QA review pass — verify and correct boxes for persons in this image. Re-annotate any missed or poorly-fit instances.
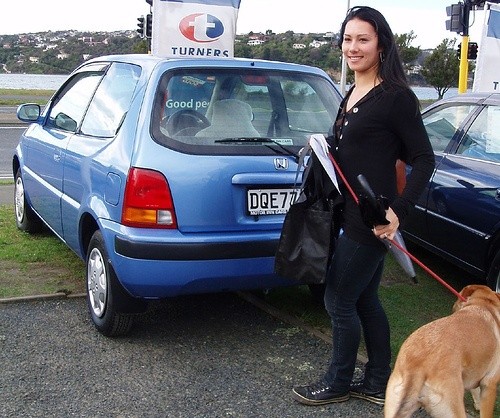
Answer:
[290,4,436,407]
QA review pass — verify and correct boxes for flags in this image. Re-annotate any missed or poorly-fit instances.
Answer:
[468,2,500,112]
[150,0,242,121]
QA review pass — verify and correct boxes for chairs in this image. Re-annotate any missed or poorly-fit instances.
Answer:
[194,98,260,140]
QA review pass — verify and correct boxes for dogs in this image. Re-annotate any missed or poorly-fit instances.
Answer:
[385,285,500,418]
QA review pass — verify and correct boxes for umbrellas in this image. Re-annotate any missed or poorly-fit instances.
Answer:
[356,174,422,285]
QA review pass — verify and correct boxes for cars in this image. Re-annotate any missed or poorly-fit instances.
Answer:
[388,94,500,297]
[11,53,345,335]
